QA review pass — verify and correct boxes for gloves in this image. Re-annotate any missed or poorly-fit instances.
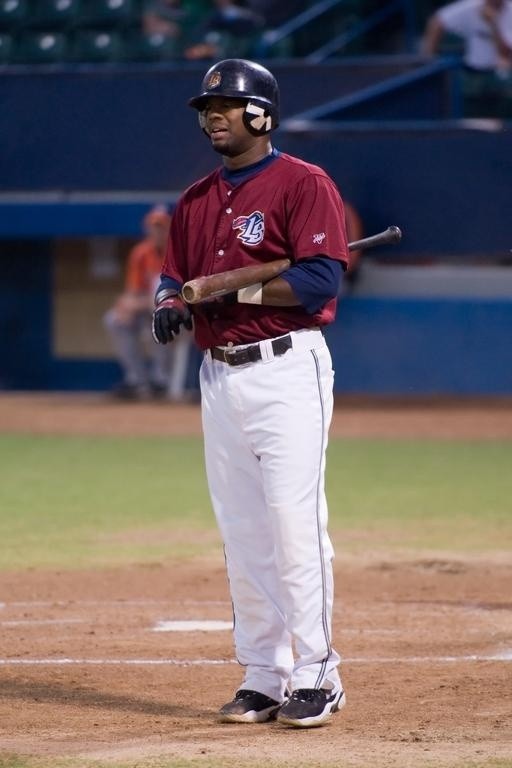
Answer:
[150,296,194,344]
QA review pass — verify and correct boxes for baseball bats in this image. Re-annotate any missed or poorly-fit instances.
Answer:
[181,225,401,304]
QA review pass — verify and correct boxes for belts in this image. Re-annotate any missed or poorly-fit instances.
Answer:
[209,334,292,365]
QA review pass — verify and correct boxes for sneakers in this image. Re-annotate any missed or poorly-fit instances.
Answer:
[277,685,346,727]
[219,686,290,727]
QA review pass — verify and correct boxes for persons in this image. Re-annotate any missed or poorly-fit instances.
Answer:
[98,200,200,409]
[131,1,238,60]
[148,55,350,730]
[415,0,512,81]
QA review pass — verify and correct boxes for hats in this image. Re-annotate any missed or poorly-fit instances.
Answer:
[145,209,172,226]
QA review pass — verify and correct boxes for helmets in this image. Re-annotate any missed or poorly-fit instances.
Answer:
[186,58,281,137]
